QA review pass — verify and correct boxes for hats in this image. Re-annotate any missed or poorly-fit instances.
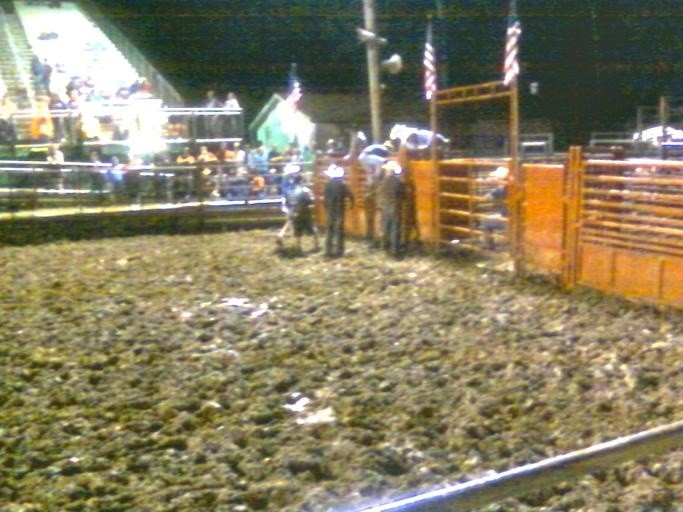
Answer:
[382,161,402,175]
[323,164,344,178]
[490,167,508,178]
[282,164,300,176]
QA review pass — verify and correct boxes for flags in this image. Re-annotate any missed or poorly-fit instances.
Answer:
[421,14,437,101]
[502,1,522,85]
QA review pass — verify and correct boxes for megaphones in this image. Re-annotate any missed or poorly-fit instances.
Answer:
[381,53,402,74]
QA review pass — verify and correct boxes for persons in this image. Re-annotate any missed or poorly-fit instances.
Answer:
[281,174,317,255]
[479,167,511,251]
[321,164,354,259]
[359,140,405,187]
[370,159,409,259]
[274,165,304,246]
[1,57,353,205]
